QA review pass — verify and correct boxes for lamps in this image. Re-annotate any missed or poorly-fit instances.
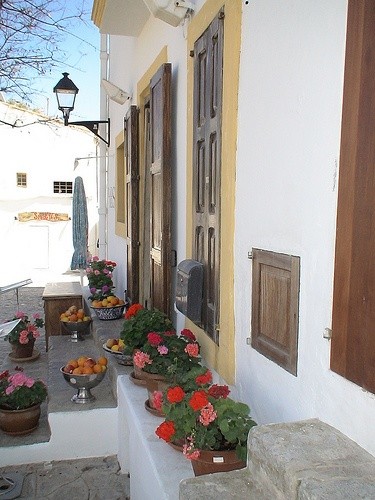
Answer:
[144,0,194,27]
[102,79,132,105]
[53,72,111,147]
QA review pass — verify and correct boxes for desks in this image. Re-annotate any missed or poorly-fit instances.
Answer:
[42,281,83,351]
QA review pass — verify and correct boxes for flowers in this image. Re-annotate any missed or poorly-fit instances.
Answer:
[120,304,257,461]
[0,366,50,409]
[3,310,45,345]
[86,256,116,299]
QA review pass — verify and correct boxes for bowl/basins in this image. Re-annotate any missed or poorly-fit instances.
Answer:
[102,342,134,366]
[89,301,128,321]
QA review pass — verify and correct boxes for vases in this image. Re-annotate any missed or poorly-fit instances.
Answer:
[169,438,184,452]
[0,404,41,435]
[129,360,162,386]
[145,377,172,417]
[191,449,245,477]
[10,338,36,358]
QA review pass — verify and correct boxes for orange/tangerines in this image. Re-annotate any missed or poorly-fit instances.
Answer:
[63,356,107,374]
[92,295,124,307]
[60,306,90,322]
[105,338,126,351]
